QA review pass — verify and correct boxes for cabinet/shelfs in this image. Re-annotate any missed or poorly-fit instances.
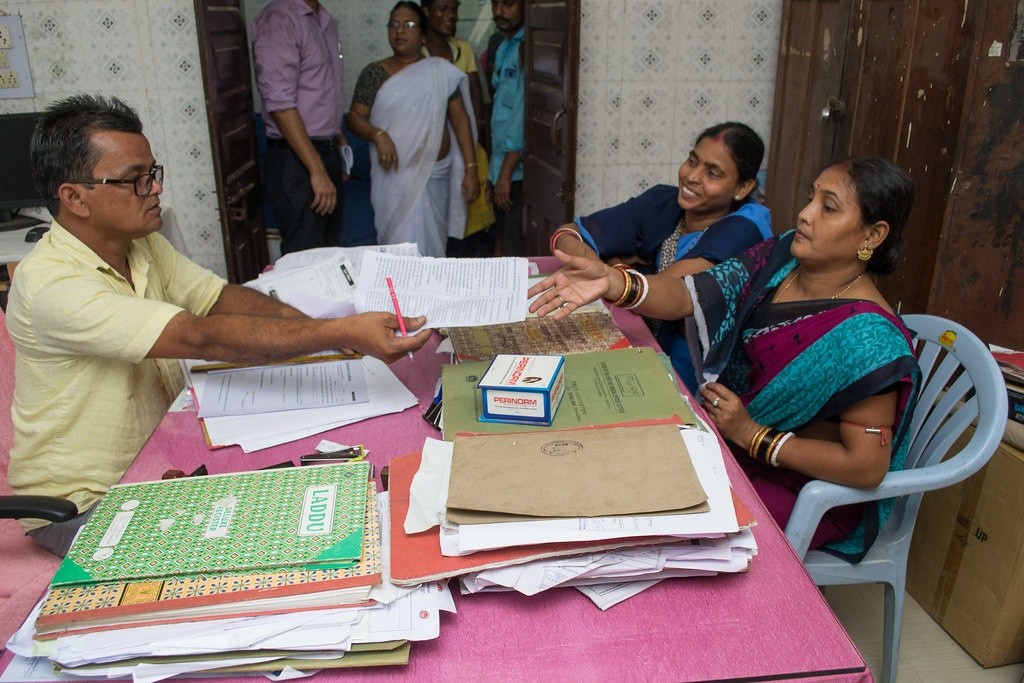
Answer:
[763,0,1024,381]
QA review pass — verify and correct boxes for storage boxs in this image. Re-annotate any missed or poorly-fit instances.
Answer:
[905,414,1024,669]
[474,353,567,427]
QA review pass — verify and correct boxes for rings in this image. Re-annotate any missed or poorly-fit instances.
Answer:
[712,396,722,407]
[556,296,565,304]
[382,155,386,160]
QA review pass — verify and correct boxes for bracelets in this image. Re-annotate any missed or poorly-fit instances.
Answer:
[602,264,649,311]
[749,427,796,467]
[549,227,583,254]
[372,129,384,140]
[463,163,478,171]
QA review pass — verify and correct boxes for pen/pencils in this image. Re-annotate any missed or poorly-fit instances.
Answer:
[386,274,415,365]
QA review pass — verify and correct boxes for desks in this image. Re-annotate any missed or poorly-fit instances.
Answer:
[0,258,877,683]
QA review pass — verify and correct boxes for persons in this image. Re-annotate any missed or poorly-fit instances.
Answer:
[6,94,432,560]
[421,0,526,258]
[549,121,776,397]
[250,0,349,257]
[345,0,481,258]
[528,151,923,564]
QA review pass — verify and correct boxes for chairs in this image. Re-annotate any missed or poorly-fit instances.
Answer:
[787,312,1009,683]
[0,306,79,651]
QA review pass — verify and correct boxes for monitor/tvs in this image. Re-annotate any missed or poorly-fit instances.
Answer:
[0,112,46,233]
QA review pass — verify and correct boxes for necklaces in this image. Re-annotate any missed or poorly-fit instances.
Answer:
[775,270,864,303]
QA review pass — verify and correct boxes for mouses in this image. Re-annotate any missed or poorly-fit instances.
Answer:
[24,227,50,243]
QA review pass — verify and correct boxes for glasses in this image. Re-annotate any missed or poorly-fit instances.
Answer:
[385,20,425,31]
[53,164,164,199]
